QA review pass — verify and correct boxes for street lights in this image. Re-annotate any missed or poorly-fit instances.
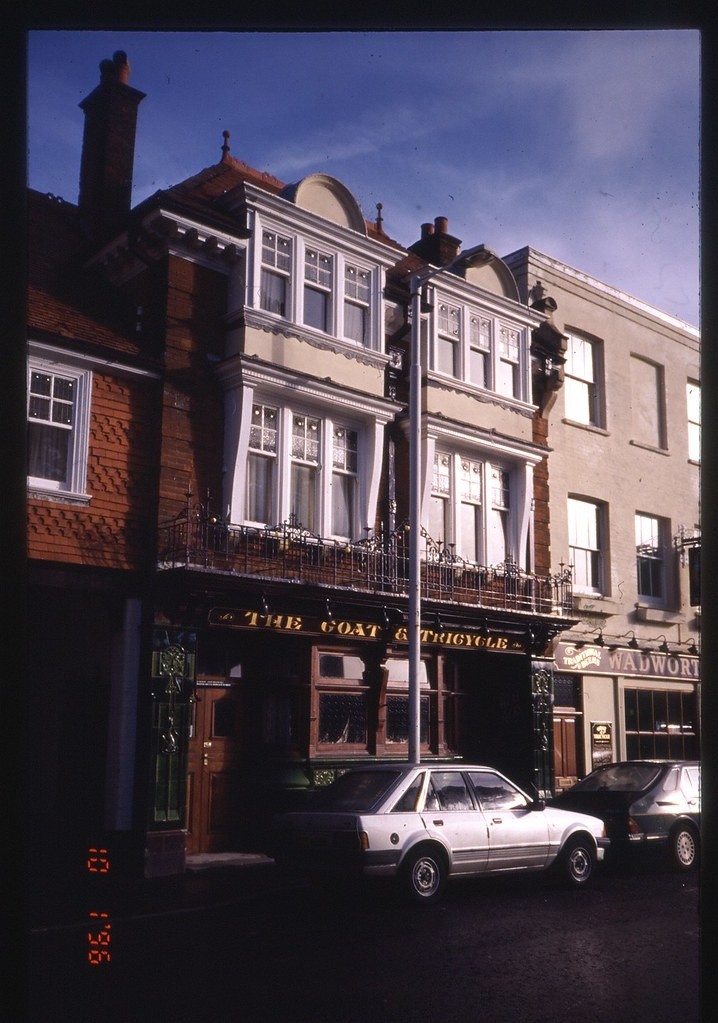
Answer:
[406,243,494,764]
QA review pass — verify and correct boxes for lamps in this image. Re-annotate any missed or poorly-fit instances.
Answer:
[620,630,639,648]
[651,634,670,653]
[681,637,698,654]
[586,627,605,647]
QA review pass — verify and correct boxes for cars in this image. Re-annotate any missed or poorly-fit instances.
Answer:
[271,763,611,907]
[548,759,702,875]
